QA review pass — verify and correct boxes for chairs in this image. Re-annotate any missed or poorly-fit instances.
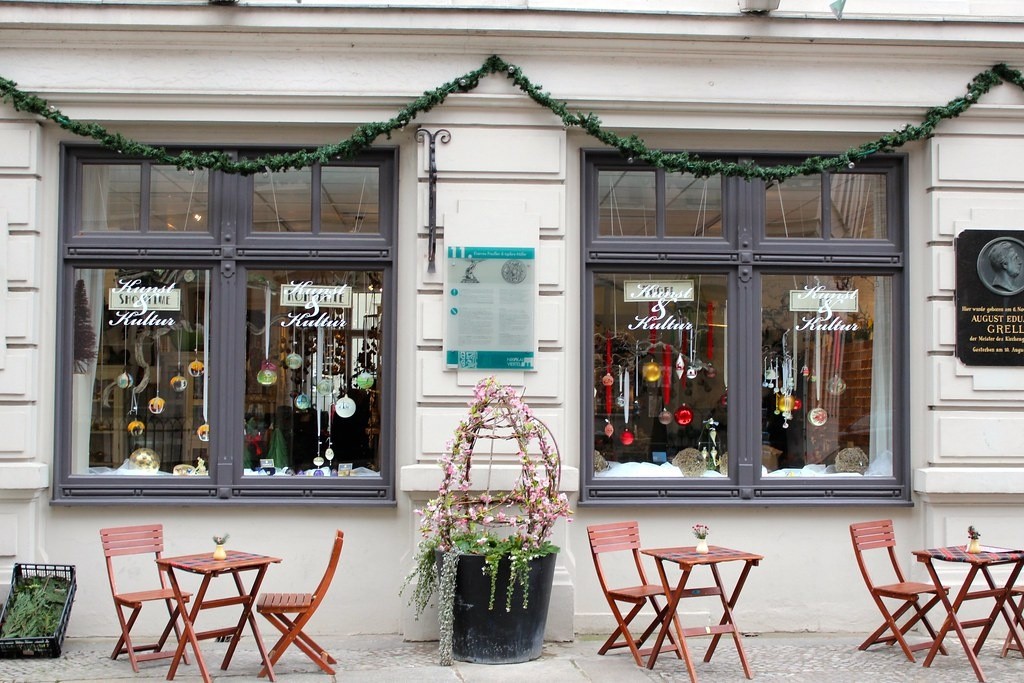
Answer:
[256,529,343,677]
[585,521,680,667]
[848,519,952,663]
[100,524,196,673]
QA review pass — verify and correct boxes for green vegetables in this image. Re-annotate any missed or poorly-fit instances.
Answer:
[0,574,72,649]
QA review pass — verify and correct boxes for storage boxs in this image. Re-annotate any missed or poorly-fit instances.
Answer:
[0,562,78,659]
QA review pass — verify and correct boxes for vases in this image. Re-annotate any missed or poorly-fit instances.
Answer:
[697,537,708,554]
[968,539,980,554]
[211,545,227,559]
[434,546,558,666]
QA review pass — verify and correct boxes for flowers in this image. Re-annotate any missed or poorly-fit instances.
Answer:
[213,532,229,544]
[396,373,578,666]
[691,523,710,539]
[967,526,982,541]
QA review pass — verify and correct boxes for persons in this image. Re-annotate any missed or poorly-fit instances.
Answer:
[988,241,1022,292]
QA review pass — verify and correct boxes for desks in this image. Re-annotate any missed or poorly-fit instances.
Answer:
[912,544,1024,683]
[155,549,283,683]
[639,545,766,683]
